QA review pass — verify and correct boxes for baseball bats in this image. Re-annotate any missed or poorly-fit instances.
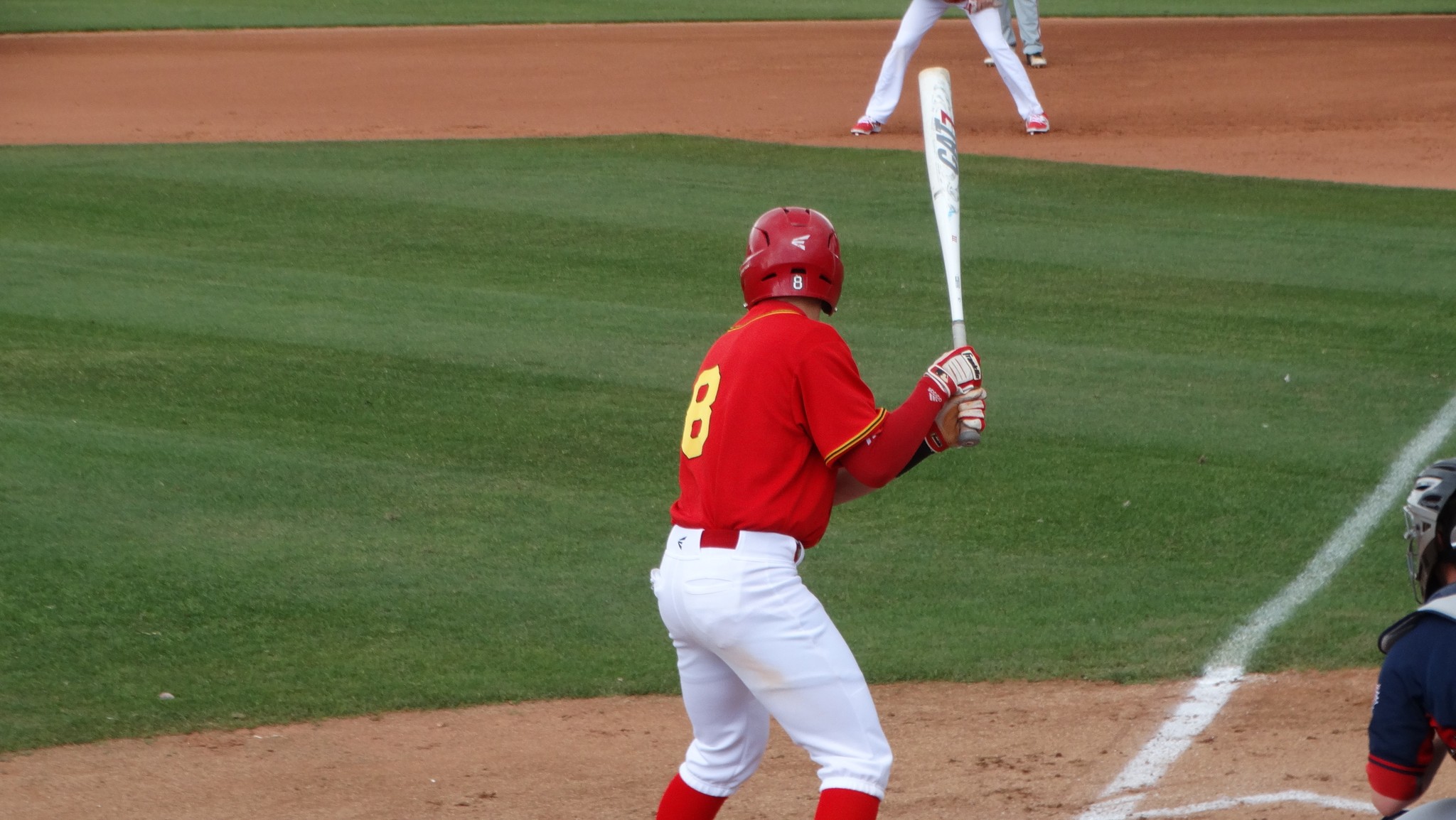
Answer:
[916,66,983,448]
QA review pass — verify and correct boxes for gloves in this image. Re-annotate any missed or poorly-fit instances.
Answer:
[925,346,982,398]
[923,387,988,454]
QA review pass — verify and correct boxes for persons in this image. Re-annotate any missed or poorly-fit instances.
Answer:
[1366,458,1456,820]
[641,207,986,820]
[847,0,1050,136]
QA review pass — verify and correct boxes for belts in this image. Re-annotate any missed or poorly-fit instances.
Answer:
[701,526,800,564]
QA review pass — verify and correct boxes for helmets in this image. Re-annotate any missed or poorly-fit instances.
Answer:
[740,208,845,316]
[1404,460,1456,598]
